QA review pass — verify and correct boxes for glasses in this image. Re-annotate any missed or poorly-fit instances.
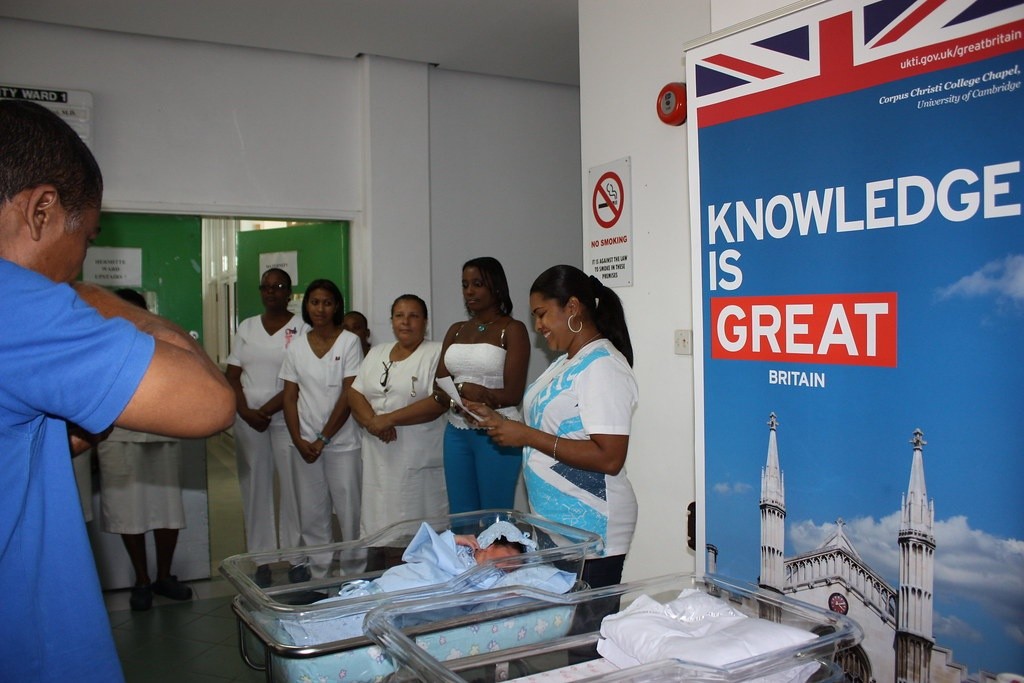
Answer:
[380,360,394,387]
[258,282,284,291]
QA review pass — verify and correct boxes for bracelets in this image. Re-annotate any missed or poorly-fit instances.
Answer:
[553,436,559,459]
[458,382,466,396]
[316,433,329,445]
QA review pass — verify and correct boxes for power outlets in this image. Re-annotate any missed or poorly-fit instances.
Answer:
[674,330,692,355]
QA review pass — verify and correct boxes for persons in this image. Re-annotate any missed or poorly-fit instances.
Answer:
[343,310,371,358]
[279,278,368,605]
[348,294,451,584]
[226,268,310,587]
[458,264,638,666]
[0,99,236,683]
[437,531,527,589]
[432,257,531,540]
[93,288,193,612]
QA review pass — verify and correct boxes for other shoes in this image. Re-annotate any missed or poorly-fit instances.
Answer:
[254,565,272,589]
[288,564,308,583]
[130,574,193,612]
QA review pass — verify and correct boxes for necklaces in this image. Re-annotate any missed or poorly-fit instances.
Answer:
[471,318,497,331]
